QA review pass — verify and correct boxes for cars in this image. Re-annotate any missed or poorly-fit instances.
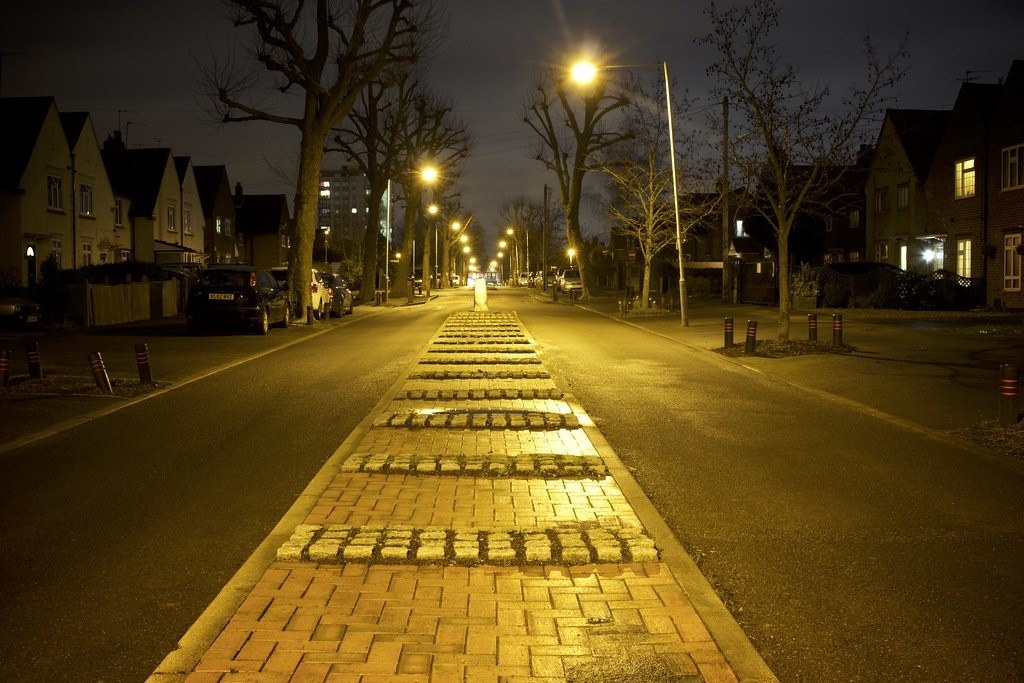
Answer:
[184,262,291,336]
[518,270,582,293]
[321,270,354,318]
[272,265,332,320]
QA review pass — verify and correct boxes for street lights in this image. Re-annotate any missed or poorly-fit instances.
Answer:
[384,169,436,302]
[411,204,439,275]
[507,228,530,287]
[567,248,574,266]
[567,57,689,328]
